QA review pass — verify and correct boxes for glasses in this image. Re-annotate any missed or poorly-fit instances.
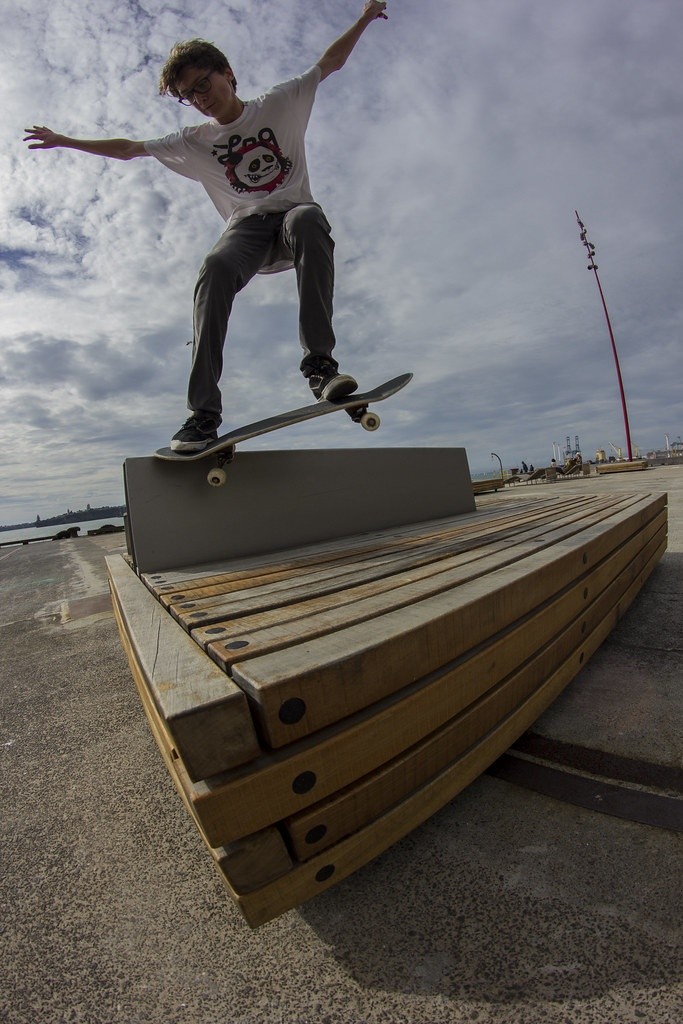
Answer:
[178,68,217,106]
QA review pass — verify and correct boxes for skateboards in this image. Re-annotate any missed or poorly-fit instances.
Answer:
[151,371,416,488]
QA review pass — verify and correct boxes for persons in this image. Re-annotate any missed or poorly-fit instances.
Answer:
[23,0,389,452]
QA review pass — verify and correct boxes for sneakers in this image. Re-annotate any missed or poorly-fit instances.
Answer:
[170,416,218,453]
[308,361,358,403]
